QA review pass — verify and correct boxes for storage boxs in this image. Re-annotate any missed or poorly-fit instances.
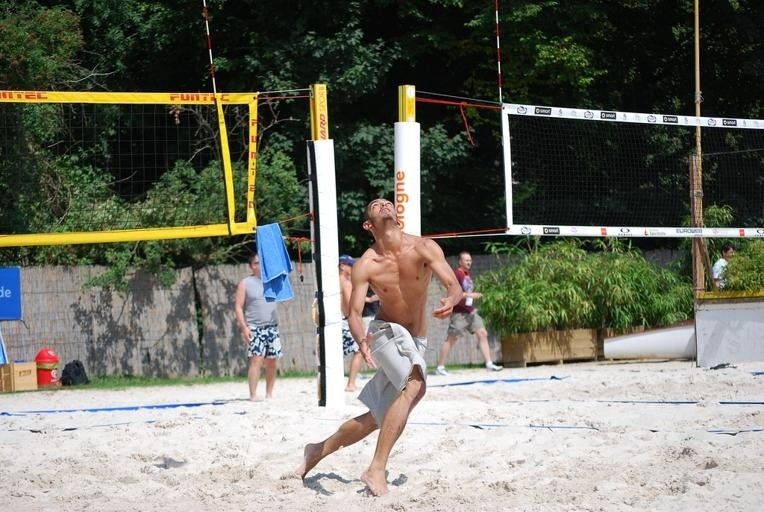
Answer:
[1,362,38,393]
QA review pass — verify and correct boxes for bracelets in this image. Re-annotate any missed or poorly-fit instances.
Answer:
[357,339,364,350]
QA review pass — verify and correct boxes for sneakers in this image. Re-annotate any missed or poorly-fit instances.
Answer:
[435,366,448,376]
[487,365,503,371]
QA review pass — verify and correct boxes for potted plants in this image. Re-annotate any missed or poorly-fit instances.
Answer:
[478,240,693,361]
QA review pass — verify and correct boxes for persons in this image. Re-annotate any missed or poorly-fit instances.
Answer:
[434,250,504,378]
[335,253,367,391]
[234,251,284,401]
[360,282,383,340]
[292,196,464,501]
[708,239,737,292]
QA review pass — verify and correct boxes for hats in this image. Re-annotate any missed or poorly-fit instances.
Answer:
[339,255,357,265]
